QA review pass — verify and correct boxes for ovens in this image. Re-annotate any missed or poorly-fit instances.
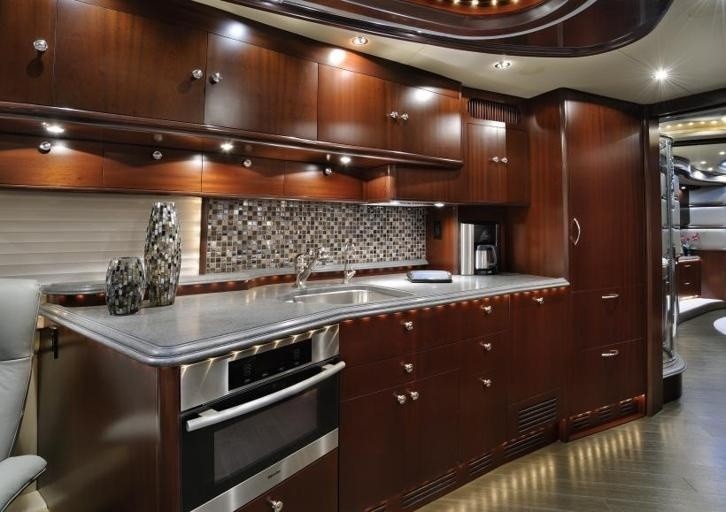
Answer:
[177,323,347,512]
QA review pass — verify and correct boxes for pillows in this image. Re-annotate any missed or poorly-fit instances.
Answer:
[0,450,48,512]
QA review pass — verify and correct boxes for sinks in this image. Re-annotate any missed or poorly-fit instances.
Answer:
[274,285,414,305]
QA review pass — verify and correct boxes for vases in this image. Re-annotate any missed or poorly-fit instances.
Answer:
[683,245,695,255]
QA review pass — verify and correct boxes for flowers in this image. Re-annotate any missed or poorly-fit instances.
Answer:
[680,232,700,246]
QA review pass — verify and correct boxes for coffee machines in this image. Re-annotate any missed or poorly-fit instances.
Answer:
[459,217,500,277]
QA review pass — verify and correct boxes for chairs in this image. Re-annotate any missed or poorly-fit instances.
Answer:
[0,277,41,512]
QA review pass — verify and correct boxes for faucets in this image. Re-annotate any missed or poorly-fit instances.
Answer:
[343,243,357,284]
[292,246,328,289]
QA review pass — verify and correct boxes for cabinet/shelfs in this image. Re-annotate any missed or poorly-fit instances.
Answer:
[318,62,461,167]
[0,0,319,148]
[340,295,510,512]
[677,259,701,296]
[566,100,648,421]
[465,118,528,204]
[513,288,568,459]
